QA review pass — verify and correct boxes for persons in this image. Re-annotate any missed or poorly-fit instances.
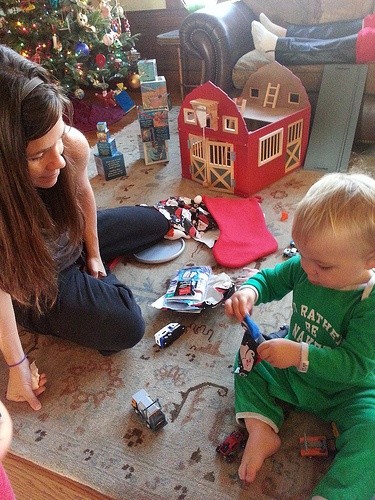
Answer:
[225,173,375,500]
[252,13,375,63]
[0,45,171,410]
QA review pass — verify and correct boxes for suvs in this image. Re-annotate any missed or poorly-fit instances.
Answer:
[215,430,248,464]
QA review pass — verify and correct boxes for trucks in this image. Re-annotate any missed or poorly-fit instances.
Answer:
[130,388,168,432]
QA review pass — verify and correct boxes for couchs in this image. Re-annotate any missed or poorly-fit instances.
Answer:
[178,0,375,145]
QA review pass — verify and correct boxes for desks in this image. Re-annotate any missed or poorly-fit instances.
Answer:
[157,30,185,102]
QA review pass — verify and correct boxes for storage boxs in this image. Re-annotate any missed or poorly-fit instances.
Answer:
[136,59,170,165]
[93,121,127,180]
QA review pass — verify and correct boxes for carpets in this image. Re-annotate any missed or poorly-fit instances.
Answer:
[0,106,375,500]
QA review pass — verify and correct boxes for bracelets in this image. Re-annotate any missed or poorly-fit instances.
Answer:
[6,355,26,367]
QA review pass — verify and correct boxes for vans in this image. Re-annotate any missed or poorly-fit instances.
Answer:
[154,322,185,349]
